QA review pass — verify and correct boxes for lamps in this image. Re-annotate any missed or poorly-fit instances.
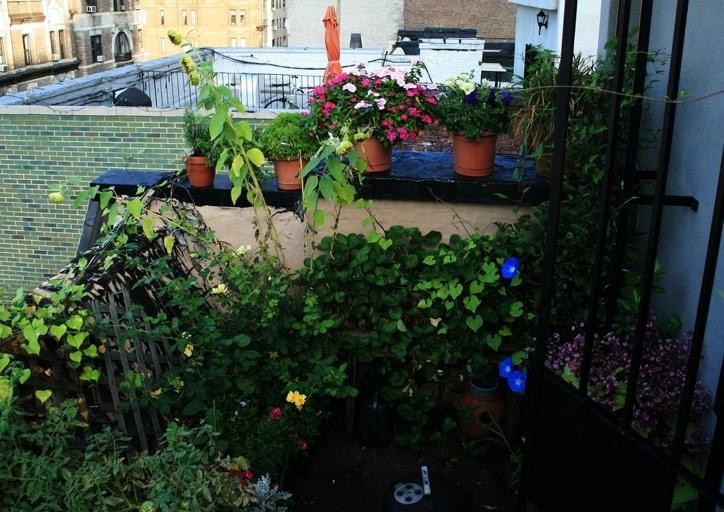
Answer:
[535,8,549,35]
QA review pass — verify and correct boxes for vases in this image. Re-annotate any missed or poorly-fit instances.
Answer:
[453,130,497,177]
[453,375,504,437]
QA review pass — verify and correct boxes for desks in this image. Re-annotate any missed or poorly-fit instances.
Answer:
[481,62,507,87]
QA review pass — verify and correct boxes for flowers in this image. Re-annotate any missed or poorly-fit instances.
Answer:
[438,72,514,129]
[302,58,436,136]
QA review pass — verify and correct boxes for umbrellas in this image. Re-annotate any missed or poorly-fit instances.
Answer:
[322,5,343,87]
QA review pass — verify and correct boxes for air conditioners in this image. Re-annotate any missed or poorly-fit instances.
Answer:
[0,64,8,73]
[135,5,141,10]
[86,6,96,13]
[120,6,125,10]
[97,56,105,62]
[52,54,60,62]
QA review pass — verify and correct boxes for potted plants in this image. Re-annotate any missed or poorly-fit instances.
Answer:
[259,112,310,191]
[361,139,392,173]
[183,101,218,187]
